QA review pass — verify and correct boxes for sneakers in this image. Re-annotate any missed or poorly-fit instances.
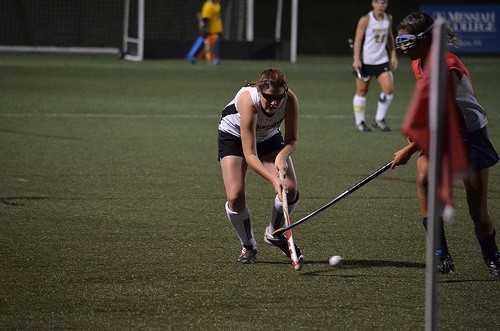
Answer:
[483,250,500,278]
[355,121,371,132]
[263,225,303,259]
[433,249,457,276]
[237,243,258,263]
[372,118,391,132]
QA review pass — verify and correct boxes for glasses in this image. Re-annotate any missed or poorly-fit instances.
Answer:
[396,32,421,51]
[260,88,287,100]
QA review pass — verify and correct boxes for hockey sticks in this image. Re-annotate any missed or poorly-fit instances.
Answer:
[197,12,212,60]
[348,38,370,81]
[272,160,394,238]
[278,172,304,271]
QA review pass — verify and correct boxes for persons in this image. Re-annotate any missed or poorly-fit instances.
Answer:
[391,12,500,276]
[197,0,222,64]
[218,68,303,263]
[353,0,398,132]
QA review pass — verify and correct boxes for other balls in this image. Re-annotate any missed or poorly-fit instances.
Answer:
[329,255,344,268]
